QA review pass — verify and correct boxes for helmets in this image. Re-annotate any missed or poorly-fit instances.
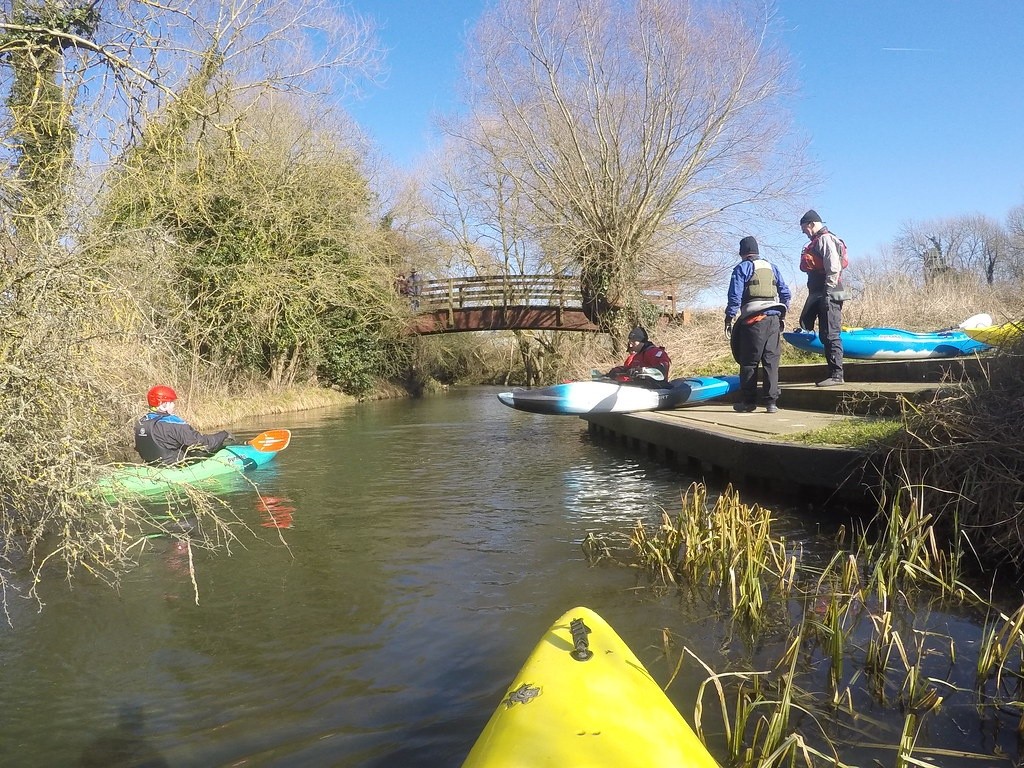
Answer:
[147,384,178,407]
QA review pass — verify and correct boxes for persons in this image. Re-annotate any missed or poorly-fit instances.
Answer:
[799,210,853,388]
[725,236,792,413]
[606,326,672,384]
[134,385,237,469]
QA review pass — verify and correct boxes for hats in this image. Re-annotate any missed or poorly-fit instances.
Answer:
[739,236,759,256]
[800,210,826,223]
[628,326,648,342]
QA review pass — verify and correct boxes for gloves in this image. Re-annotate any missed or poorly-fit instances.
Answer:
[225,431,236,442]
[780,319,786,335]
[609,366,625,380]
[625,368,642,380]
[724,315,733,340]
[815,285,835,313]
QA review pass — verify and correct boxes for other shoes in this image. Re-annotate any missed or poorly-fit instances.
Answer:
[733,399,756,413]
[815,375,845,386]
[766,403,780,412]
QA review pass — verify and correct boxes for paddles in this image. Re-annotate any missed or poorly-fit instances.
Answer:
[224,429,292,452]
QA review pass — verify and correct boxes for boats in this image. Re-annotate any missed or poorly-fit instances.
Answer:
[783,313,994,361]
[83,444,278,512]
[460,608,727,768]
[495,375,742,417]
[965,315,1024,349]
